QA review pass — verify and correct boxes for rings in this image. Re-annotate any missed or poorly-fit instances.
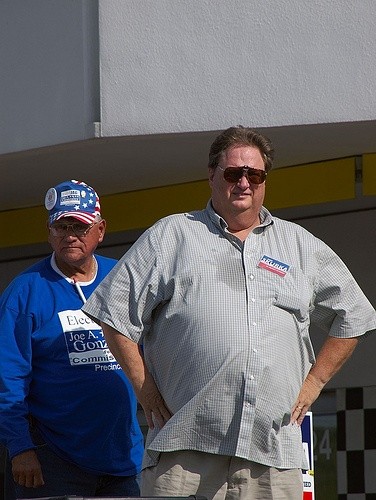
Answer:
[296,405,303,410]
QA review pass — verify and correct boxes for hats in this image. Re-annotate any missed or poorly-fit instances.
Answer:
[46,179,102,227]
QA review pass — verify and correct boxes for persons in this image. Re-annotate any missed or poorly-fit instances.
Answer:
[80,125,376,500]
[0,178,150,500]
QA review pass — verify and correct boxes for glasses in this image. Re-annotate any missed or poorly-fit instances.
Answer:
[216,165,268,185]
[48,219,101,233]
